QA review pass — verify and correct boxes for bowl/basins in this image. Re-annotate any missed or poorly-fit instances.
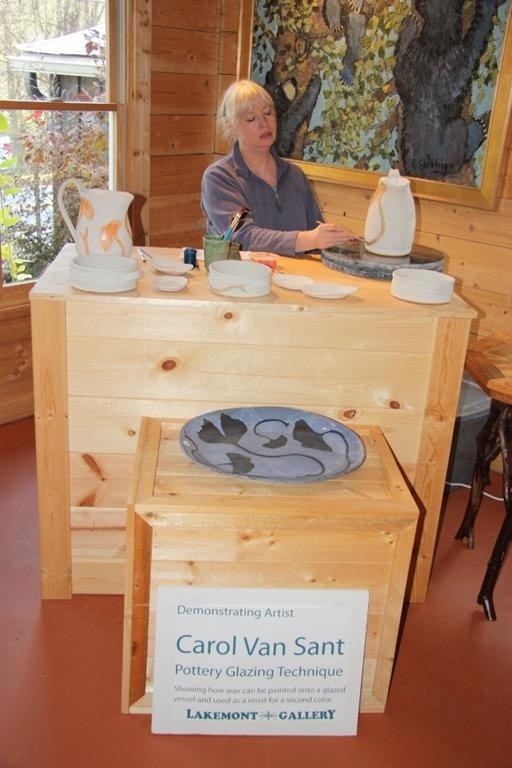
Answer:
[390,268,456,306]
[70,254,140,292]
[208,260,273,298]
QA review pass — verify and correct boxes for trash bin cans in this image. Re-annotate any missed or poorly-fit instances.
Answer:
[444,369,492,494]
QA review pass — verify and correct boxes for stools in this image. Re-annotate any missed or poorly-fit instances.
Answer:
[454,374,510,623]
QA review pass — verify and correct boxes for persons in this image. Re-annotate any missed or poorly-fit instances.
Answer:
[200,78,355,258]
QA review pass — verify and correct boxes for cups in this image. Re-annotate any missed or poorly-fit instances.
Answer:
[184,249,196,269]
[202,236,229,272]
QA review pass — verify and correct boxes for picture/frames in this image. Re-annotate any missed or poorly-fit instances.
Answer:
[234,0,512,213]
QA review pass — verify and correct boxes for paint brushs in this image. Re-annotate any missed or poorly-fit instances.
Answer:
[136,247,152,262]
[316,220,365,242]
[221,207,250,240]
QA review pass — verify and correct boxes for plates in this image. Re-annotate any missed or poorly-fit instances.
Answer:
[304,282,358,300]
[178,407,366,484]
[151,259,193,274]
[152,274,188,291]
[271,274,314,292]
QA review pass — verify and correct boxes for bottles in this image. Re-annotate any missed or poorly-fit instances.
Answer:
[364,168,416,257]
[228,242,241,260]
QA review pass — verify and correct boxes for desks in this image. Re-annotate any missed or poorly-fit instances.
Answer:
[25,241,485,605]
[115,412,423,722]
